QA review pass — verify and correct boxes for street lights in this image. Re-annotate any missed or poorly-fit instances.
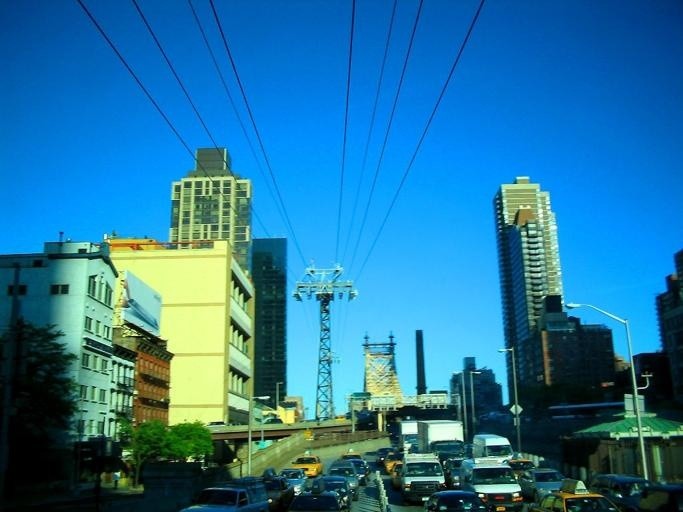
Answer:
[247,396,270,476]
[497,346,521,451]
[566,302,648,481]
[276,381,283,412]
[453,371,468,442]
[469,370,483,433]
[75,367,115,493]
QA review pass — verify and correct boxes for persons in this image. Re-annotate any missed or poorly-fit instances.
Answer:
[587,500,602,512]
[629,483,640,497]
